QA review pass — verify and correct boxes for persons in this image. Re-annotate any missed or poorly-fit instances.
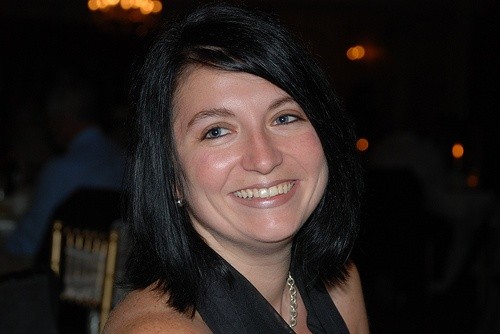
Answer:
[97,6,373,334]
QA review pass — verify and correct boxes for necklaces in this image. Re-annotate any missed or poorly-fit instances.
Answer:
[284,269,298,330]
